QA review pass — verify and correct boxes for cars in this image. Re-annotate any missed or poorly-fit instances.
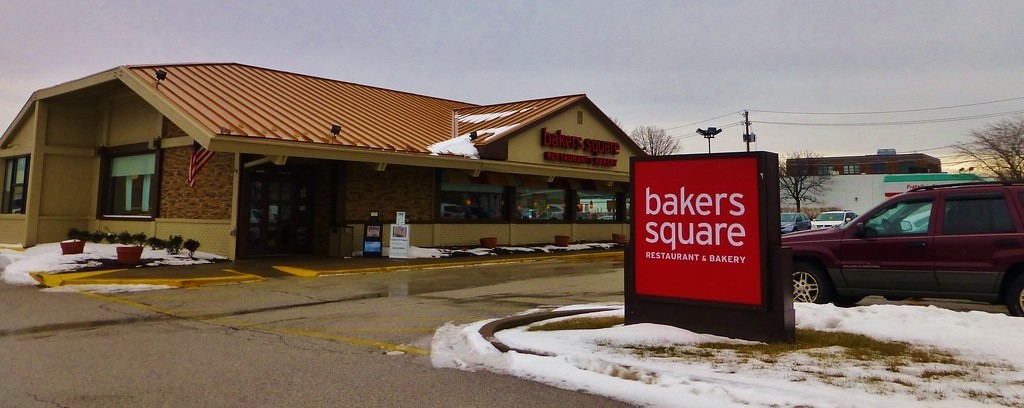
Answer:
[441,202,616,221]
[781,212,811,234]
[899,206,950,233]
[810,209,860,230]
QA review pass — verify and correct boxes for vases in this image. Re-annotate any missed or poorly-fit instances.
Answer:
[612,234,626,243]
[61,241,85,254]
[480,237,497,248]
[555,236,570,248]
[116,246,144,266]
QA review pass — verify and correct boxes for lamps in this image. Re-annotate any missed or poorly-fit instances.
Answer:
[156,70,167,88]
[968,167,974,174]
[590,201,593,208]
[6,143,22,148]
[330,124,341,137]
[959,167,965,174]
[469,131,477,141]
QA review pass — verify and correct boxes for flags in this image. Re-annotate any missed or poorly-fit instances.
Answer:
[188,140,216,188]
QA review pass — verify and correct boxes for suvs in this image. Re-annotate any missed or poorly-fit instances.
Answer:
[779,178,1024,318]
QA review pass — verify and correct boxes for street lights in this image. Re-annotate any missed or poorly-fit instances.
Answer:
[695,126,722,153]
